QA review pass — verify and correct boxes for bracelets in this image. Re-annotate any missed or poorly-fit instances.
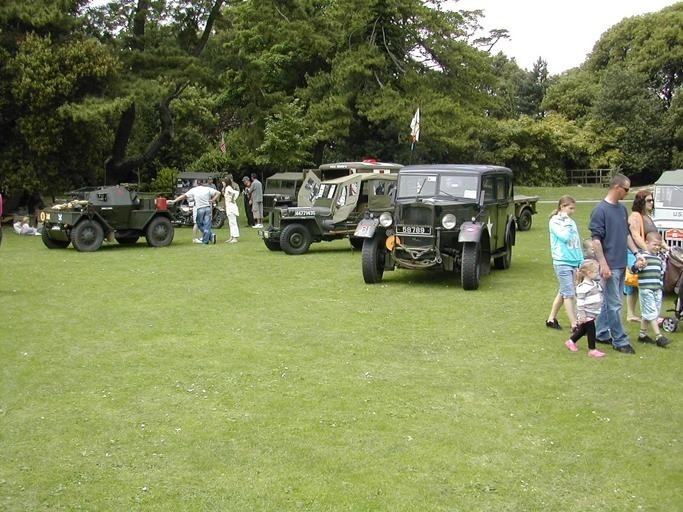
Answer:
[633,251,640,256]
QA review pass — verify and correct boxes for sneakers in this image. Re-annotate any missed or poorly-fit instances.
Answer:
[247,224,263,229]
[626,316,642,322]
[613,343,635,354]
[596,337,612,344]
[571,326,579,333]
[192,234,240,244]
[656,336,673,346]
[546,318,562,330]
[638,335,655,344]
[565,340,578,352]
[588,349,605,357]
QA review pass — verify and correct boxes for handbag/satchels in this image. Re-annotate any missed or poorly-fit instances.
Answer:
[625,265,640,287]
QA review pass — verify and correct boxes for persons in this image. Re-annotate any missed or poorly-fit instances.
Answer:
[631,232,674,346]
[20,217,37,235]
[588,173,647,354]
[581,238,596,260]
[623,190,676,325]
[166,172,263,244]
[12,219,42,236]
[565,259,611,357]
[545,195,585,333]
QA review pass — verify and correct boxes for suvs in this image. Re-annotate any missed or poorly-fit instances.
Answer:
[646,168,682,256]
[259,157,540,292]
[173,171,221,201]
[35,185,176,252]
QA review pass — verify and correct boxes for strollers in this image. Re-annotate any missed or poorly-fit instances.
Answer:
[661,246,682,335]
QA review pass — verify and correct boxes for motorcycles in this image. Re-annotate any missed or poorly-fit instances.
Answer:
[171,200,225,229]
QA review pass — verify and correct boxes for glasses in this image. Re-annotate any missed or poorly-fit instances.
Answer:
[646,199,654,202]
[619,187,629,193]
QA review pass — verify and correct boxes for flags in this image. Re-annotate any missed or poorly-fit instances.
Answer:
[410,107,420,142]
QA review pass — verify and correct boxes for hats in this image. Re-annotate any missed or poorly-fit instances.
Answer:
[241,176,250,181]
